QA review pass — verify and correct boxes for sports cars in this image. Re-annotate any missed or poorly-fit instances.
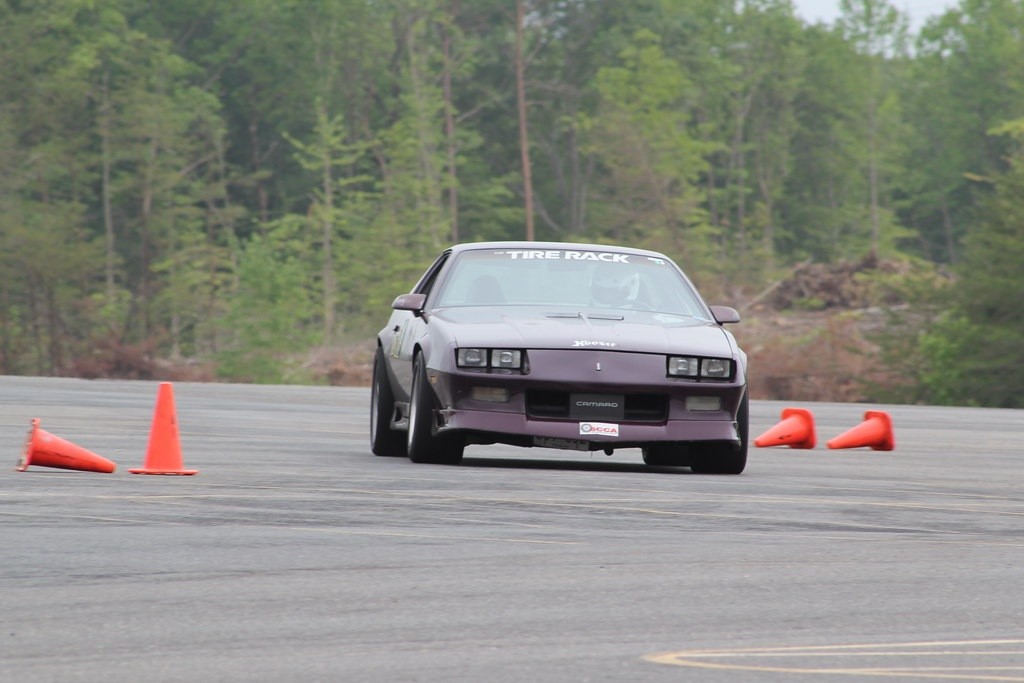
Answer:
[368,238,752,477]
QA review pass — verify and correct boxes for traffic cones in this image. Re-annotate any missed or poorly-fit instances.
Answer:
[754,406,818,449]
[827,410,896,452]
[14,417,117,473]
[125,380,199,477]
